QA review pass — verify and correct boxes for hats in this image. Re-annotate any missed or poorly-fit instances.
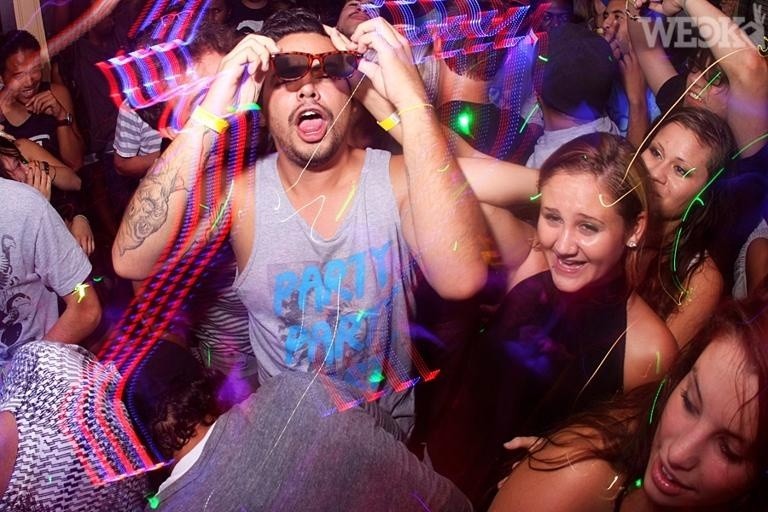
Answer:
[531,23,617,120]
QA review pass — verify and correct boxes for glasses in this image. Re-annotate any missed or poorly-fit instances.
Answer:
[268,51,364,82]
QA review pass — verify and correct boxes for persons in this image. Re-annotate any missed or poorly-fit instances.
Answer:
[0,1,768,512]
